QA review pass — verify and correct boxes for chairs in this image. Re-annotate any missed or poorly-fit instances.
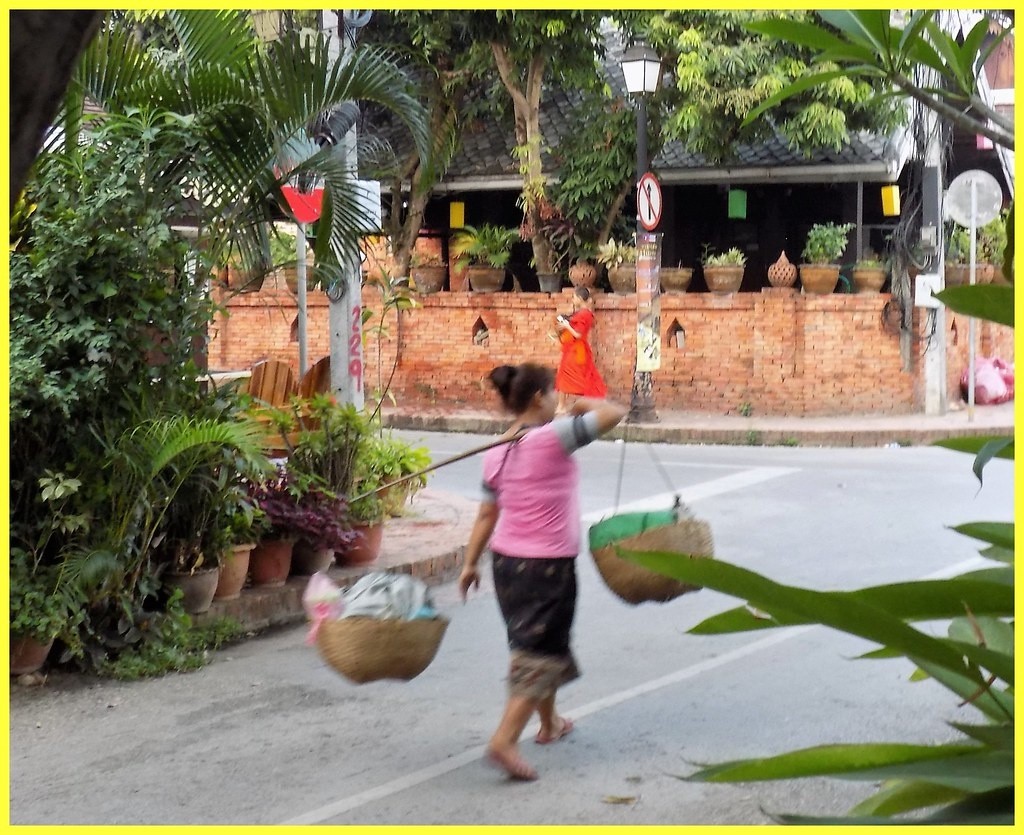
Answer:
[246,356,330,457]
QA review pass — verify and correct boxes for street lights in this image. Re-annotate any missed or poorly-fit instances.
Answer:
[619,44,665,422]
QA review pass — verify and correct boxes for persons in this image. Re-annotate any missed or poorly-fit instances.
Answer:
[553,285,608,410]
[459,362,623,778]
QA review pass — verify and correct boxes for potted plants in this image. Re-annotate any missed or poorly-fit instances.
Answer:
[222,230,316,291]
[407,250,448,294]
[853,255,887,293]
[447,222,522,292]
[530,251,566,292]
[598,237,637,292]
[10,389,433,679]
[568,242,598,287]
[702,246,747,293]
[940,222,1008,288]
[798,222,856,293]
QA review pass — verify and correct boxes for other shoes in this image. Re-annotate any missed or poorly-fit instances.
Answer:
[483,746,542,781]
[536,718,573,743]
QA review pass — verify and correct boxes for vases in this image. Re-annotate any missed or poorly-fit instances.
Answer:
[659,267,693,292]
